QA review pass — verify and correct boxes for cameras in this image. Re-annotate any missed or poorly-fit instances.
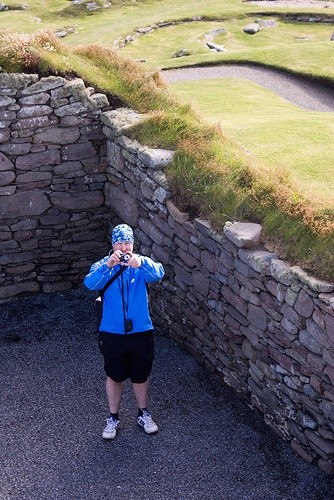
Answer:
[119,254,131,263]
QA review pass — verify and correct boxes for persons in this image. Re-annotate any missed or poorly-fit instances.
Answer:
[83,224,165,439]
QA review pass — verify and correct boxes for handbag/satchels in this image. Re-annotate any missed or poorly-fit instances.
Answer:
[96,295,112,326]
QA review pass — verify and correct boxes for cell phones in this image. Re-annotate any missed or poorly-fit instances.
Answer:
[125,319,133,332]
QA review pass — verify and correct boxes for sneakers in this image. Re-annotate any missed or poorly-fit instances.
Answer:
[102,416,121,440]
[136,412,159,435]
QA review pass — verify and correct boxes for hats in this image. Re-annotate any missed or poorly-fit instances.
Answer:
[111,224,134,245]
